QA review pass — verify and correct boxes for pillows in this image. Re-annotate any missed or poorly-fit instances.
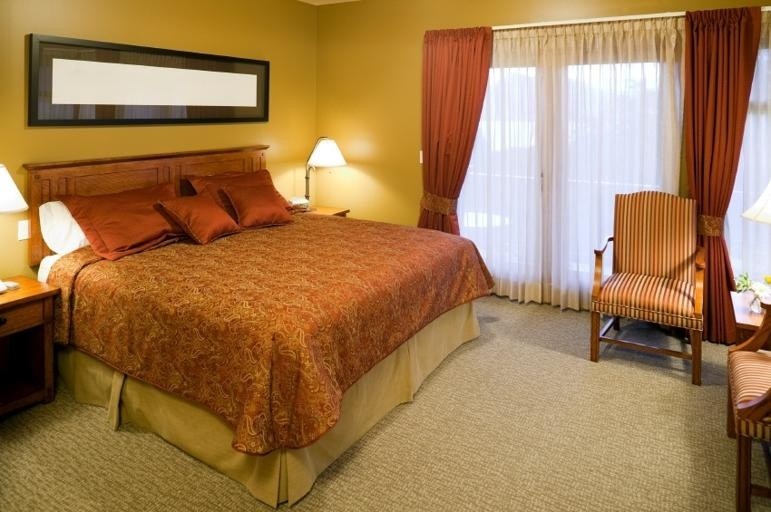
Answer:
[220,184,293,230]
[186,170,294,225]
[157,196,242,245]
[38,200,91,256]
[57,184,188,261]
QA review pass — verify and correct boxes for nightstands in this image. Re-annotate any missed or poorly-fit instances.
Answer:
[0,275,62,420]
[306,203,350,218]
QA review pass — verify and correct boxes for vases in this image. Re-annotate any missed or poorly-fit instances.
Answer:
[749,291,767,314]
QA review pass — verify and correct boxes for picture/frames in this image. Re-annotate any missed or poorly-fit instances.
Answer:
[27,33,270,125]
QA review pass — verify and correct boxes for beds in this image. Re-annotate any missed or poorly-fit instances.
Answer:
[24,144,497,507]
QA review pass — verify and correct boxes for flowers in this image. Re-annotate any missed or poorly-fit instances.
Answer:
[733,272,771,304]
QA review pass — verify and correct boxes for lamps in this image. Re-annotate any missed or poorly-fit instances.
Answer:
[742,184,771,307]
[0,164,29,295]
[304,136,347,214]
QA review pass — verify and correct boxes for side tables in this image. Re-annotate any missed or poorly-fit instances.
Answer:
[732,290,766,330]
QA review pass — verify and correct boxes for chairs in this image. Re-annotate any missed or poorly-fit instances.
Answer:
[726,301,771,511]
[593,190,705,385]
